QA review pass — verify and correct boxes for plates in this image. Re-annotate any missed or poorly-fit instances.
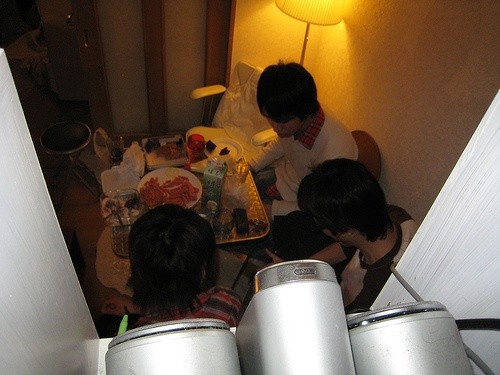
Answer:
[204,137,243,163]
[137,167,203,209]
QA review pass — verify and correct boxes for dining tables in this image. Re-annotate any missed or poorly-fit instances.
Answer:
[111,140,269,288]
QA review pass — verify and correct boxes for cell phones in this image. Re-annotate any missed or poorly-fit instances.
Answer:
[233,208,250,234]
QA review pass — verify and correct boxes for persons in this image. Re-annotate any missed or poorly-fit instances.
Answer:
[242,158,419,314]
[95,203,243,339]
[216,62,358,287]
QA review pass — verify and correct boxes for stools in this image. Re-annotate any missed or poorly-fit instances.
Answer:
[39,121,102,202]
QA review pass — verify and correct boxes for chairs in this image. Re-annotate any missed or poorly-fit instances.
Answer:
[350,129,382,185]
[185,60,285,162]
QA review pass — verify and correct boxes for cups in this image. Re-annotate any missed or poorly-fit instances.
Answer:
[101,187,142,259]
[212,208,236,236]
[187,134,206,162]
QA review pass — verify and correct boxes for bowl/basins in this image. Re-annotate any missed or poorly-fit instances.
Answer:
[141,134,189,171]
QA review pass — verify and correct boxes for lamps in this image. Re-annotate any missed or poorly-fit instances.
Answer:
[273,0,346,67]
